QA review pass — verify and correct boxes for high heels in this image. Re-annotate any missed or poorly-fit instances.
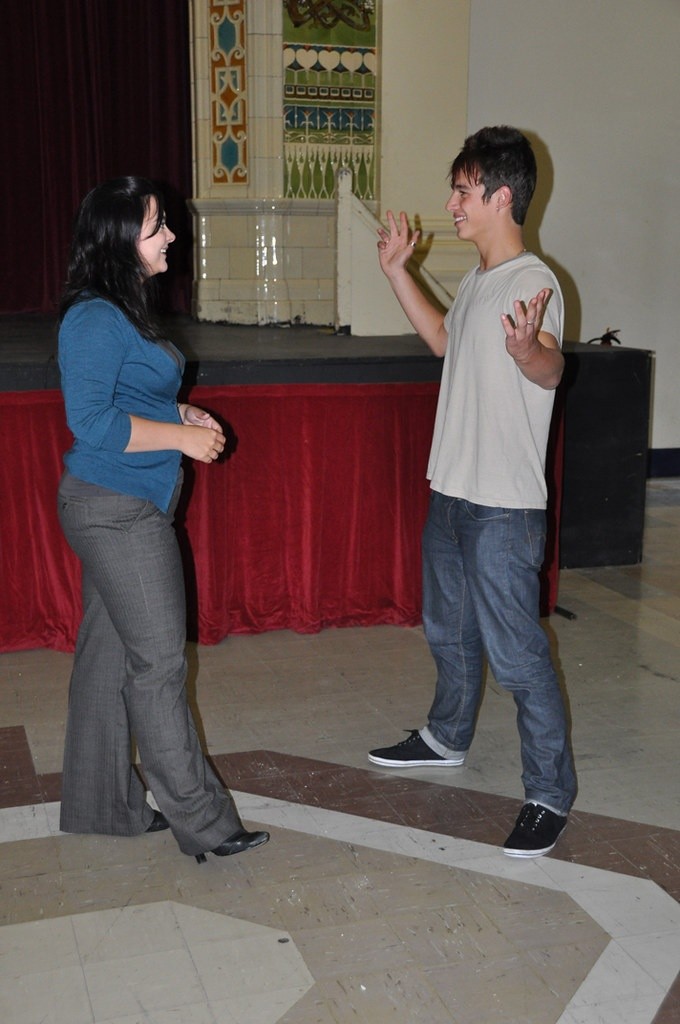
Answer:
[146,809,169,833]
[196,829,271,865]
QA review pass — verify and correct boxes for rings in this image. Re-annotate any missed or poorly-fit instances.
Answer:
[408,241,416,247]
[384,240,390,244]
[525,320,535,325]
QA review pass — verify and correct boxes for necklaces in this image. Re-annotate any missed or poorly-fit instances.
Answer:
[518,248,528,254]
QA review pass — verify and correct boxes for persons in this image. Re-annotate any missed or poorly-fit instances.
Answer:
[370,124,578,859]
[55,174,271,863]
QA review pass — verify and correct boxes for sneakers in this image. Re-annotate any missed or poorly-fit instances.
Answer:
[503,803,568,857]
[368,728,465,767]
